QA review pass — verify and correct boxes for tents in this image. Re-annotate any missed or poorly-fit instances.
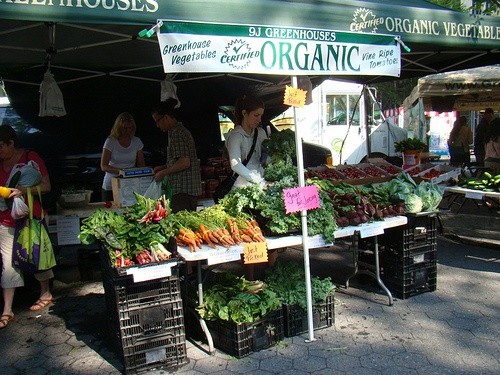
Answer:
[0,0,500,342]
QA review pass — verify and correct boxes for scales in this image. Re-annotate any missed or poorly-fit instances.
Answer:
[119,167,155,178]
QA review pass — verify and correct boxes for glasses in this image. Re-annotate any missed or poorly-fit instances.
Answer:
[153,115,165,126]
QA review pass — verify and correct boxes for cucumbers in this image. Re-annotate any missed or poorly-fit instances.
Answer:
[462,171,500,191]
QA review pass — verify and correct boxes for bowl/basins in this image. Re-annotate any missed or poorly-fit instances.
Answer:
[62,189,93,206]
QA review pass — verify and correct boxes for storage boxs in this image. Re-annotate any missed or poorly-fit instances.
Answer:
[207,293,337,358]
[358,215,438,300]
[308,159,462,186]
[77,241,189,375]
[111,175,154,206]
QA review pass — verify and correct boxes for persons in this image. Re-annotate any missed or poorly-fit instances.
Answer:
[100,112,145,202]
[152,104,201,214]
[225,95,268,191]
[0,124,54,327]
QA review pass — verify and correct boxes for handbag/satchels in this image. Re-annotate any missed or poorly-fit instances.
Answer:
[6,160,42,189]
[11,194,30,220]
[213,176,235,205]
[142,177,164,201]
[11,185,57,273]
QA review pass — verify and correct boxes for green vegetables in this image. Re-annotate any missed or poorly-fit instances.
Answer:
[261,128,305,181]
[218,178,338,244]
[394,137,429,152]
[77,192,183,269]
[195,261,336,323]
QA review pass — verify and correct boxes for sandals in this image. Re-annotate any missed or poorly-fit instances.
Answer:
[29,297,55,311]
[0,313,14,329]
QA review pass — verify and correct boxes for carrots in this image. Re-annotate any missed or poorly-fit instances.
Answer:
[176,219,266,253]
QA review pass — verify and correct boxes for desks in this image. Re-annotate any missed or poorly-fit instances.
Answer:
[444,186,500,215]
[176,216,410,354]
[44,201,113,245]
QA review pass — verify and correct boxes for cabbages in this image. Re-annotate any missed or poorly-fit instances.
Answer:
[372,173,443,213]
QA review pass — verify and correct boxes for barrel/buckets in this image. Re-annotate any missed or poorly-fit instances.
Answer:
[402,150,420,165]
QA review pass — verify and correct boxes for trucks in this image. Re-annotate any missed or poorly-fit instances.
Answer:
[218,76,409,168]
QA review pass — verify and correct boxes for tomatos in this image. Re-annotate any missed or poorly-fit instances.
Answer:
[311,164,452,179]
